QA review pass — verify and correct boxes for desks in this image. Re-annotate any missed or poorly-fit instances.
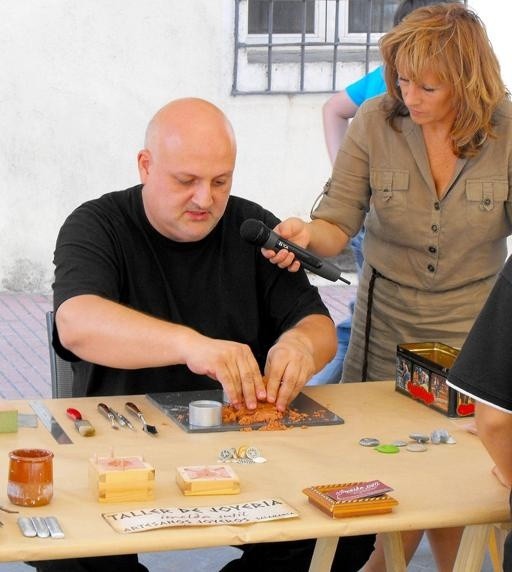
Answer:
[0,380,512,572]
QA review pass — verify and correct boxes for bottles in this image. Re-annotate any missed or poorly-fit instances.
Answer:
[5,447,56,510]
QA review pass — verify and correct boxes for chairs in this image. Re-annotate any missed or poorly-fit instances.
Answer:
[46,312,74,399]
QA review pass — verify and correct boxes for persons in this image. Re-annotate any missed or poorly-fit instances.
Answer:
[302,0,459,384]
[446,252,512,572]
[24,98,377,572]
[258,0,511,572]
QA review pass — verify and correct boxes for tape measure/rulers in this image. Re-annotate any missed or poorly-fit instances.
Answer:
[29,398,73,444]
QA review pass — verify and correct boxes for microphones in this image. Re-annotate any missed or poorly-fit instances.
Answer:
[240,218,341,281]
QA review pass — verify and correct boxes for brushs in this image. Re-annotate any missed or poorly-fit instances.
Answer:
[67,408,95,437]
